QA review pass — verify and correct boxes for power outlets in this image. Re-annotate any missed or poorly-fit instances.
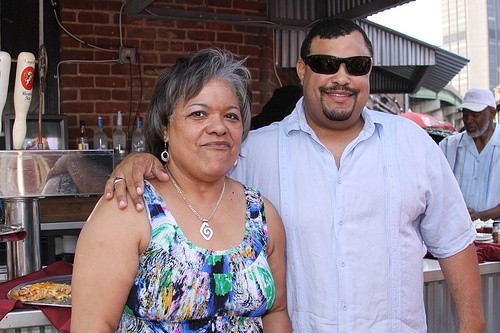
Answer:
[119,46,136,64]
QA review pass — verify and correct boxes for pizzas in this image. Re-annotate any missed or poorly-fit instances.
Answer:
[10,282,71,303]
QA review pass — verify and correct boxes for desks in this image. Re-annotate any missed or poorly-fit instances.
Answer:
[0,258,500,333]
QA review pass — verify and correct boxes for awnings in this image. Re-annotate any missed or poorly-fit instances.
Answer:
[265,0,470,95]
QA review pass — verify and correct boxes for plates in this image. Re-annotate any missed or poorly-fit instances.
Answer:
[474,232,494,243]
[7,274,72,308]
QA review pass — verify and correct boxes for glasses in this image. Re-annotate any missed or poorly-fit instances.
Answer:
[304,55,372,75]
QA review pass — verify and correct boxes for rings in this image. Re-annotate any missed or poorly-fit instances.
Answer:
[113,178,123,184]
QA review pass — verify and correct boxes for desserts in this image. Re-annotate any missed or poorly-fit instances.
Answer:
[472,218,494,234]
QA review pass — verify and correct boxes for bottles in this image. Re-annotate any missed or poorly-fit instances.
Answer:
[131,116,145,152]
[113,111,127,150]
[492,222,500,243]
[93,116,108,150]
[75,120,89,150]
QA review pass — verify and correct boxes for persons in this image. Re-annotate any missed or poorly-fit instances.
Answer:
[41,151,112,194]
[105,17,486,333]
[70,49,293,333]
[439,87,500,221]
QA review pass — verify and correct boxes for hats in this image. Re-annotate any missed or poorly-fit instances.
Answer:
[456,88,496,112]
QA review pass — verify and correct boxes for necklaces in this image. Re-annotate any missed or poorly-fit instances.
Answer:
[165,164,226,240]
